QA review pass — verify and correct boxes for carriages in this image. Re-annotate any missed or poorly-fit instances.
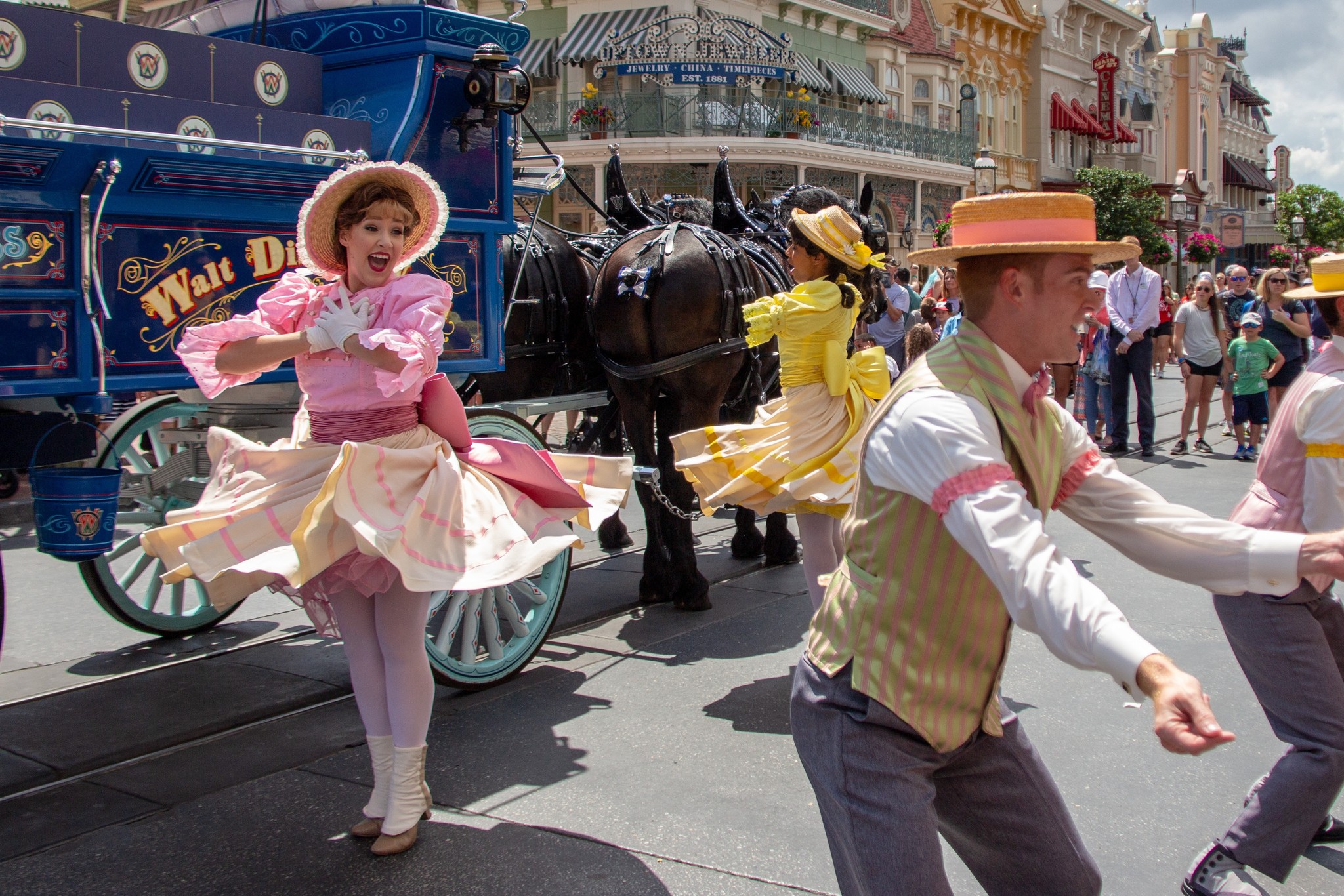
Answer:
[0,0,889,687]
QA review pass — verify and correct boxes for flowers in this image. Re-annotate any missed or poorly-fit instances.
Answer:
[1265,244,1298,268]
[571,82,616,126]
[776,87,820,131]
[1181,232,1225,266]
[1138,230,1179,267]
[932,213,954,248]
[1300,245,1328,268]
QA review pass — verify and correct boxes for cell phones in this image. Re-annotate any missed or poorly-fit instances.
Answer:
[881,271,891,288]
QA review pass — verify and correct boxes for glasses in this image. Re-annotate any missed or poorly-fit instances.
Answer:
[1267,278,1287,285]
[1288,279,1295,283]
[1195,285,1211,293]
[1243,323,1258,328]
[1185,287,1192,290]
[1298,271,1306,274]
[1230,276,1248,281]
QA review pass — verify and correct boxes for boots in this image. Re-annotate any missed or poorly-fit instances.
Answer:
[352,733,395,836]
[373,744,434,855]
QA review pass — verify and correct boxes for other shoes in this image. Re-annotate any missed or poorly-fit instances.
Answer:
[1219,416,1275,443]
[1153,354,1186,383]
[1243,773,1343,843]
[1087,429,1155,456]
[1181,839,1264,896]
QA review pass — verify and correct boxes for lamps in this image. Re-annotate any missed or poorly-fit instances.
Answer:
[899,220,914,252]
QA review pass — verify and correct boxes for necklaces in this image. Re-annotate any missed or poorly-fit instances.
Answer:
[1195,300,1208,309]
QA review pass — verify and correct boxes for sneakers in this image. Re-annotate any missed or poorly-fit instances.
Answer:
[1242,444,1258,460]
[1233,444,1246,459]
[1170,440,1189,455]
[1193,437,1211,453]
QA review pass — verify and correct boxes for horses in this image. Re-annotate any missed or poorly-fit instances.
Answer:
[484,195,710,548]
[585,175,891,609]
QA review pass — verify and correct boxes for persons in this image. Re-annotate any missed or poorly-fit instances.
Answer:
[790,192,1344,896]
[95,390,198,467]
[1151,251,1338,435]
[1226,313,1285,461]
[854,255,964,384]
[540,410,581,443]
[465,380,483,407]
[1181,252,1344,896]
[671,203,891,612]
[1171,276,1229,455]
[1100,236,1160,456]
[1045,261,1114,447]
[141,159,633,855]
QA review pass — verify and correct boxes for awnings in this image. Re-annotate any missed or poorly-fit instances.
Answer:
[1243,105,1273,116]
[765,52,834,97]
[1223,152,1281,193]
[1230,79,1271,107]
[551,6,668,68]
[1050,91,1137,144]
[815,58,890,106]
[513,38,559,79]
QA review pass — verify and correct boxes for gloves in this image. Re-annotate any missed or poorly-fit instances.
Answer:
[314,286,371,354]
[303,296,375,355]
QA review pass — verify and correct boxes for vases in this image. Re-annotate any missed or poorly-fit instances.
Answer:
[784,120,802,139]
[586,122,608,140]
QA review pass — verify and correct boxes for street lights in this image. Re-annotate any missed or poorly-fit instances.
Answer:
[1288,212,1305,270]
[1170,186,1189,295]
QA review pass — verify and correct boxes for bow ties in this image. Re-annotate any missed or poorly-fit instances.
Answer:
[1024,367,1051,415]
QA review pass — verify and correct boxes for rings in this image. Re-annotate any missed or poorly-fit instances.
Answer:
[1090,320,1092,322]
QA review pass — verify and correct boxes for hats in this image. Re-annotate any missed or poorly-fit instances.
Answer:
[1283,270,1301,285]
[908,191,1144,266]
[792,204,887,270]
[1281,253,1344,300]
[931,300,953,313]
[1085,271,1110,290]
[296,161,450,282]
[1241,312,1263,326]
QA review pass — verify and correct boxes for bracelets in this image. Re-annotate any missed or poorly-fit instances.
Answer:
[912,275,917,278]
[1170,302,1175,306]
[1179,358,1186,366]
[886,295,888,300]
